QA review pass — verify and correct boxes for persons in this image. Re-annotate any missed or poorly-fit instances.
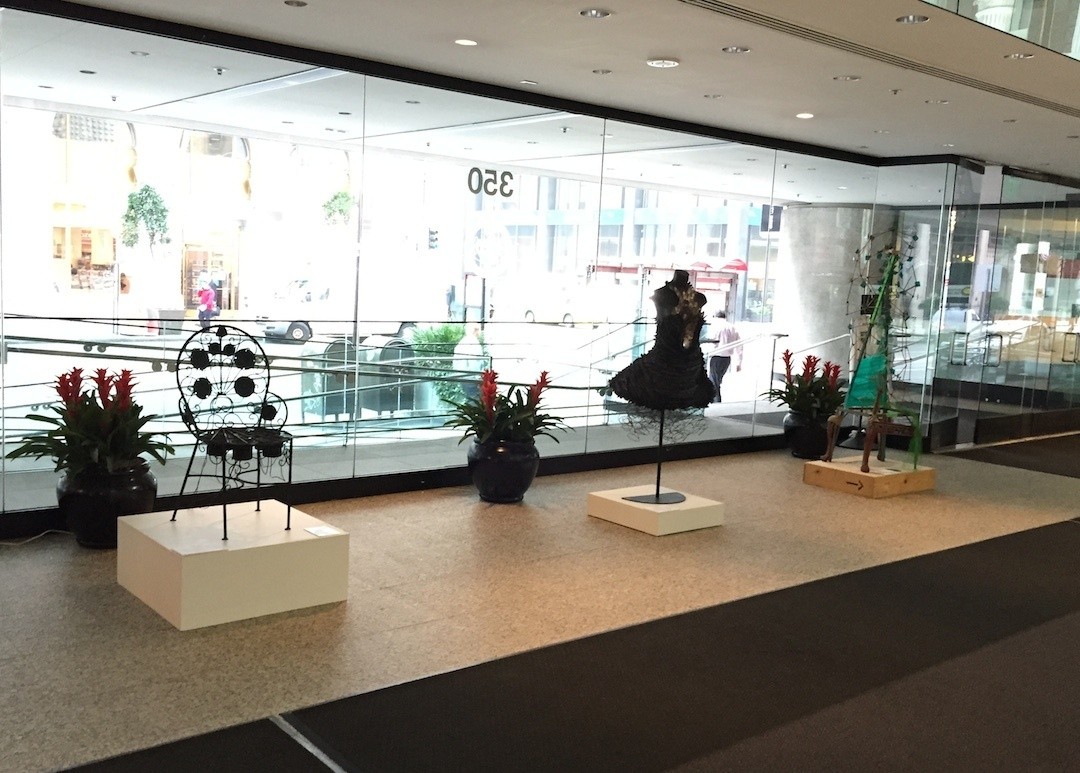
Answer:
[196,280,215,332]
[706,311,742,405]
[606,269,721,441]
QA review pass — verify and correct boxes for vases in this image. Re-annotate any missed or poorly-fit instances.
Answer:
[782,410,830,460]
[55,461,158,548]
[468,438,540,503]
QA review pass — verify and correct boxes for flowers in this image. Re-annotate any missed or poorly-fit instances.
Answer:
[757,348,848,419]
[440,357,576,444]
[5,367,175,476]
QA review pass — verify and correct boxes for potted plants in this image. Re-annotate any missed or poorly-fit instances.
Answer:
[413,323,465,417]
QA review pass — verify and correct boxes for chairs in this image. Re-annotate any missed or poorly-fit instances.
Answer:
[170,325,294,540]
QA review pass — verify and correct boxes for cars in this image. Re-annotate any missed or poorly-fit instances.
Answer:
[515,272,618,324]
[253,267,424,344]
